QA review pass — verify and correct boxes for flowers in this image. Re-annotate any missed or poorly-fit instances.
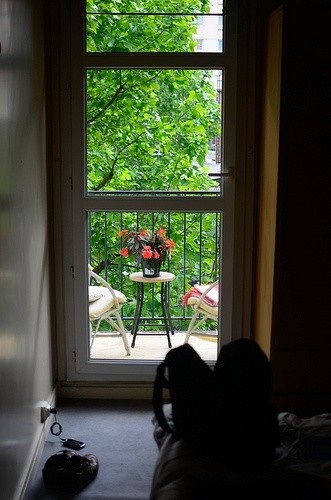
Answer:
[119,227,176,271]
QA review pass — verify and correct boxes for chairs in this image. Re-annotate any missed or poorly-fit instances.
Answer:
[88,264,132,356]
[184,278,219,346]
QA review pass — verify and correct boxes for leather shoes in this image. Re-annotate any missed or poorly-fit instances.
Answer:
[42,450,98,500]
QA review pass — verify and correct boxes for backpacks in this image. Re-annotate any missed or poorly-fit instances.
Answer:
[151,338,283,470]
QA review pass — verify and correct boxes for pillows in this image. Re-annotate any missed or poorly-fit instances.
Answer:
[89,286,103,303]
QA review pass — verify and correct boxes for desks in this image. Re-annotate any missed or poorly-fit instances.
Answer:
[129,271,176,348]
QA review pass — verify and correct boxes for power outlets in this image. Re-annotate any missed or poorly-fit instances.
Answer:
[41,401,51,423]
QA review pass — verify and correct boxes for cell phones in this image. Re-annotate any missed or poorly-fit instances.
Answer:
[63,439,85,449]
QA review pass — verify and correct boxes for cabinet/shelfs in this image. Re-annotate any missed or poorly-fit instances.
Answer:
[251,0,331,422]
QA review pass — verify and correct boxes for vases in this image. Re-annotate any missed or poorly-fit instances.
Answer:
[141,258,162,278]
